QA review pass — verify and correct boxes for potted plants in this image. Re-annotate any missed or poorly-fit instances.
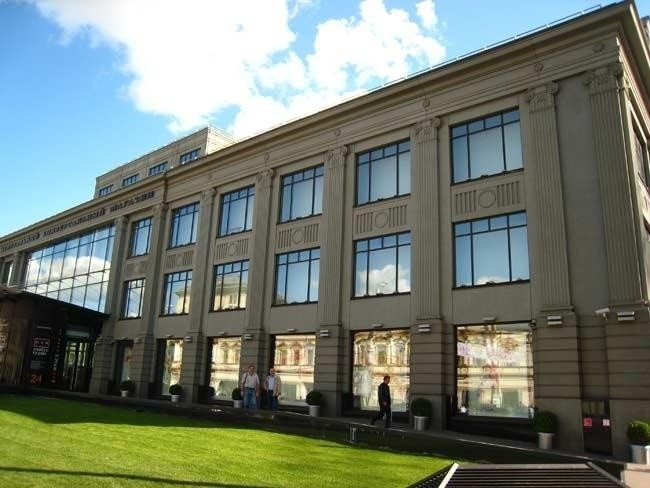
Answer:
[411,398,432,433]
[306,391,323,417]
[120,380,131,397]
[232,388,243,408]
[533,410,559,451]
[625,419,649,464]
[169,384,183,403]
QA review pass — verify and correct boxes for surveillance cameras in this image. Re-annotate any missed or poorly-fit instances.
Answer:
[595,307,610,315]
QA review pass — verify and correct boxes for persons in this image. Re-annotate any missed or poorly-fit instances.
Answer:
[241,365,260,409]
[372,376,391,428]
[264,367,281,411]
